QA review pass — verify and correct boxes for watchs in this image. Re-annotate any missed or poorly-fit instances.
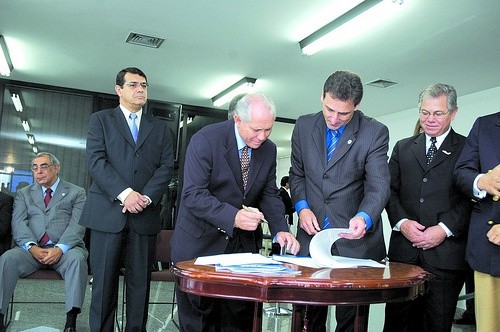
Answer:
[28,243,36,249]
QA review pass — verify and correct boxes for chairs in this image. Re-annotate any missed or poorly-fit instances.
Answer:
[1,230,173,332]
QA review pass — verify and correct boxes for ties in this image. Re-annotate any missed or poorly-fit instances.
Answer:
[426,136,437,167]
[38,188,53,247]
[129,113,139,144]
[324,130,339,229]
[240,144,251,191]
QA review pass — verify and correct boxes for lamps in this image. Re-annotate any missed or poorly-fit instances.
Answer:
[212,77,257,107]
[8,89,38,154]
[0,35,13,76]
[299,0,385,55]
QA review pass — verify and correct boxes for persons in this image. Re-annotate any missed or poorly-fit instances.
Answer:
[77,66,175,332]
[382,81,473,332]
[453,260,477,325]
[415,118,425,135]
[454,112,500,332]
[0,151,88,332]
[227,94,248,120]
[288,70,392,332]
[267,175,297,256]
[171,92,300,332]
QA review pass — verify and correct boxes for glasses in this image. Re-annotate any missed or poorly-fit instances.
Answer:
[419,107,450,119]
[31,164,53,172]
[124,83,149,90]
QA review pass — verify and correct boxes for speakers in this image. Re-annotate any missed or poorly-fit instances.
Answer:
[146,99,182,161]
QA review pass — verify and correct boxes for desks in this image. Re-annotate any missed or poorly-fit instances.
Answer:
[175,254,425,332]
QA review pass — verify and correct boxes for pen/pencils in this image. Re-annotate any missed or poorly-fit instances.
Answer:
[242,205,268,223]
[120,204,124,206]
